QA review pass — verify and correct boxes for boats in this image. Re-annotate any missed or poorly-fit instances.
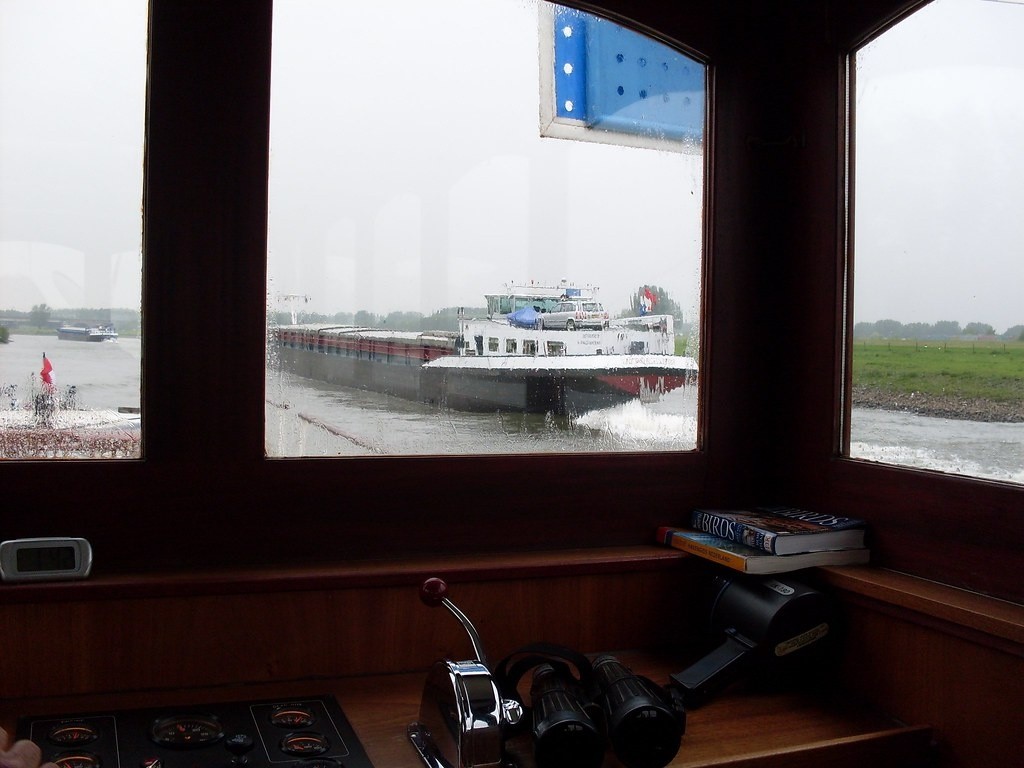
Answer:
[267,280,699,420]
[56,323,118,342]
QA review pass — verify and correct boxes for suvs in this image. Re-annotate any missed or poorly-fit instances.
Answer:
[537,301,610,332]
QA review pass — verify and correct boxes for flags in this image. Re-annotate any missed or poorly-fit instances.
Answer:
[639,288,657,316]
[40,357,57,396]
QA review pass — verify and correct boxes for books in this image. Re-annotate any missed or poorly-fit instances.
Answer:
[688,506,866,555]
[657,527,869,576]
